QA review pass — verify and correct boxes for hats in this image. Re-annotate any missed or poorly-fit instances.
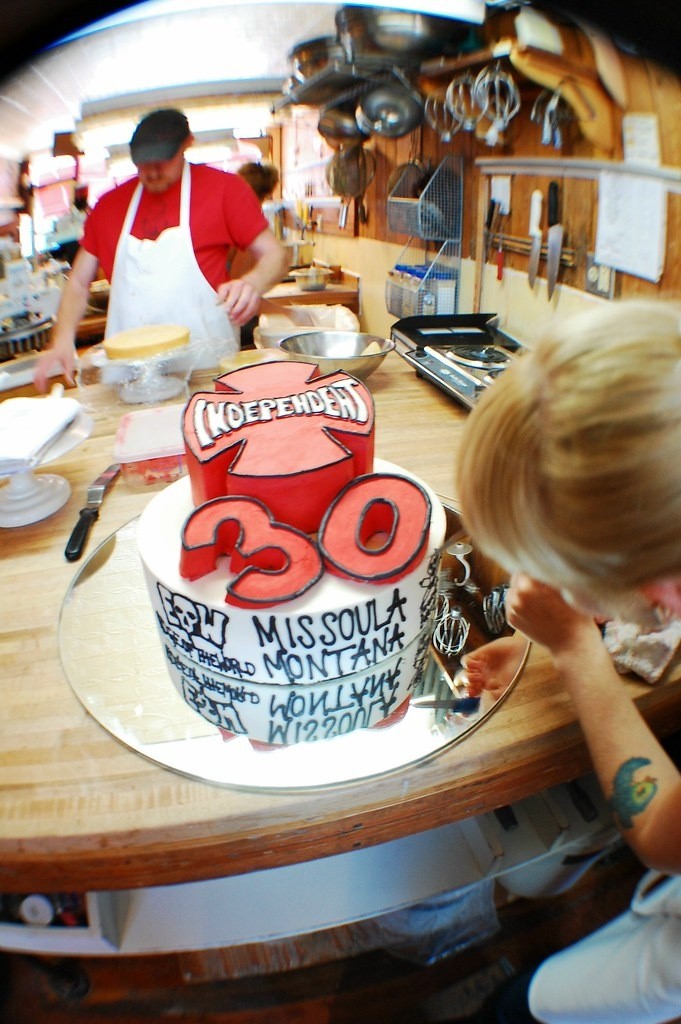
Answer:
[128,109,190,165]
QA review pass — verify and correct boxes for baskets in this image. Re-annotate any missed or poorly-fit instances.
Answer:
[385,235,461,320]
[387,153,463,241]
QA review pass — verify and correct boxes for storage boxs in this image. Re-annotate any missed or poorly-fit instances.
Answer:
[109,403,188,493]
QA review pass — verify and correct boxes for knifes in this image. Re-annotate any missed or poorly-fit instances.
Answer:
[527,189,543,289]
[547,181,563,302]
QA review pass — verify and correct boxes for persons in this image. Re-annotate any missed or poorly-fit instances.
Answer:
[32,106,289,392]
[448,300,681,1024]
[227,160,281,350]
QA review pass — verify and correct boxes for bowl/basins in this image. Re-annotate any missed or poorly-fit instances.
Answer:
[277,330,396,381]
[288,262,334,291]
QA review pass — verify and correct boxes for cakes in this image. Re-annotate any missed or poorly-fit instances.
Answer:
[135,360,447,744]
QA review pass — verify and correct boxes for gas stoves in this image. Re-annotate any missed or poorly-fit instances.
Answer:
[389,313,533,411]
